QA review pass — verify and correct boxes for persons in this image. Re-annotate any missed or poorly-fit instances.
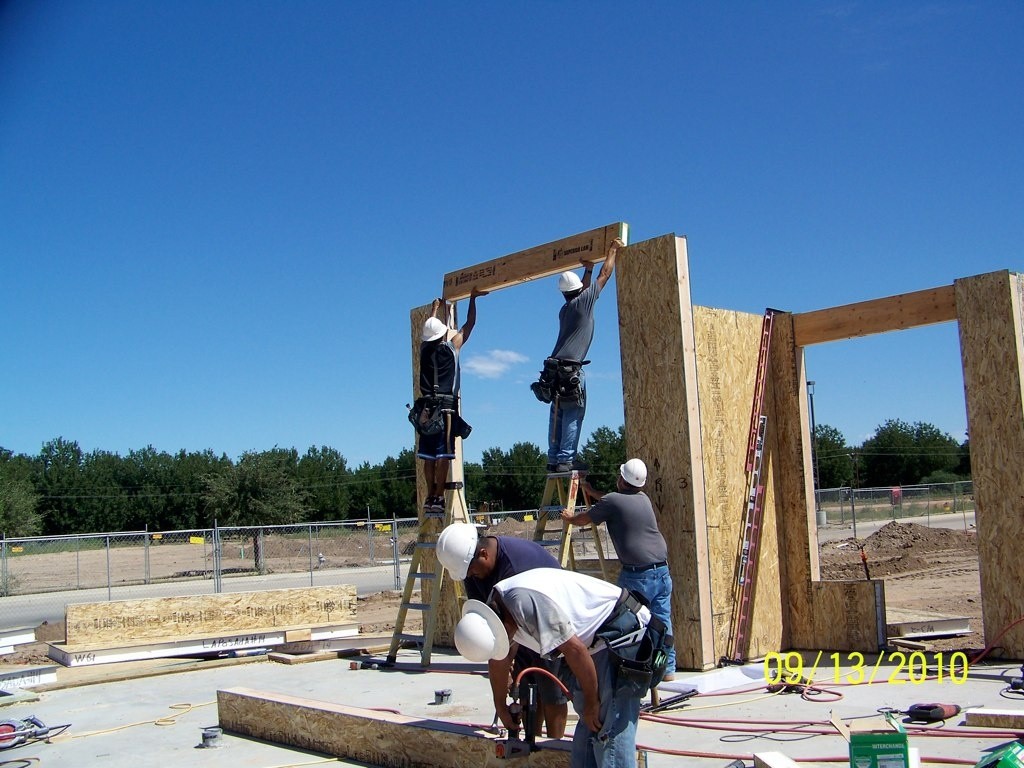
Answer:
[544,235,627,475]
[558,457,677,683]
[452,564,650,768]
[436,522,568,743]
[409,284,492,519]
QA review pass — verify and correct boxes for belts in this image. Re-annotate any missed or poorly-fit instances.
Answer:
[622,561,669,576]
[547,356,590,367]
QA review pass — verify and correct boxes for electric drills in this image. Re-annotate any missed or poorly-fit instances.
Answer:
[492,698,547,761]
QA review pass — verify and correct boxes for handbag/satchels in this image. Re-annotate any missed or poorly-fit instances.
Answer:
[610,615,676,703]
[410,396,443,434]
[531,360,585,409]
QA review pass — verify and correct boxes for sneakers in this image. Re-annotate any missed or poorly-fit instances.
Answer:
[557,461,590,471]
[548,464,558,472]
[431,494,445,513]
[423,496,433,513]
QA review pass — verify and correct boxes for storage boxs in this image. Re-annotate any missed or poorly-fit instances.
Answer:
[972,740,1024,768]
[828,710,921,768]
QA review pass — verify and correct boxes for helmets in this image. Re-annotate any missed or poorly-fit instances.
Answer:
[420,317,448,342]
[436,522,488,582]
[558,271,583,292]
[455,600,510,662]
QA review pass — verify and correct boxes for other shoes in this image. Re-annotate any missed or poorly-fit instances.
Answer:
[662,673,676,682]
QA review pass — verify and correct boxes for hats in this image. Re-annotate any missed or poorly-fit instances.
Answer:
[619,458,648,488]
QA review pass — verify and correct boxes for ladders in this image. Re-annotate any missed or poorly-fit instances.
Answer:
[386,478,473,671]
[527,468,611,586]
